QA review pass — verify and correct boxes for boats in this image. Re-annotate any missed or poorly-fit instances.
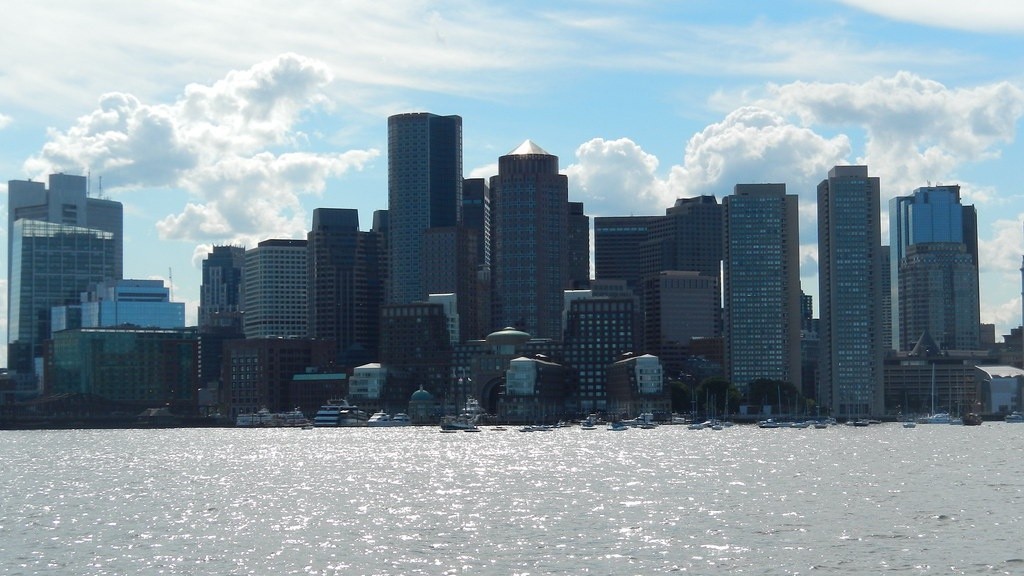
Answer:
[532,424,548,431]
[962,412,983,425]
[491,426,507,431]
[687,418,882,431]
[302,426,314,429]
[439,430,457,433]
[518,425,533,432]
[1004,415,1024,423]
[917,413,954,423]
[640,422,657,429]
[903,422,917,428]
[580,426,597,430]
[543,424,561,428]
[464,426,481,432]
[441,421,478,430]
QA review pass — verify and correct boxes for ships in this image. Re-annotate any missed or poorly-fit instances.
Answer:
[619,412,686,425]
